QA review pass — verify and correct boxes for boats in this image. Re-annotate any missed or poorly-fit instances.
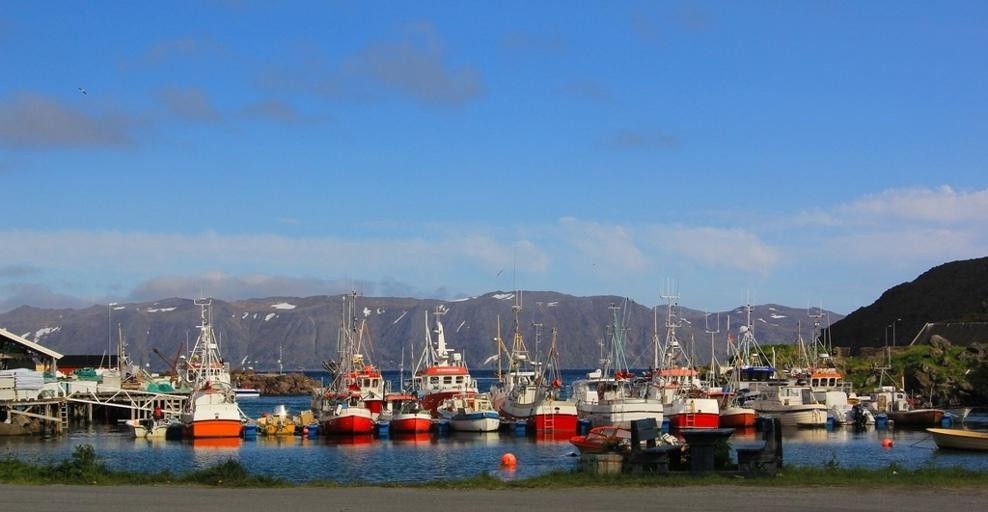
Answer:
[95,303,136,378]
[567,426,690,459]
[924,427,987,452]
[720,426,934,453]
